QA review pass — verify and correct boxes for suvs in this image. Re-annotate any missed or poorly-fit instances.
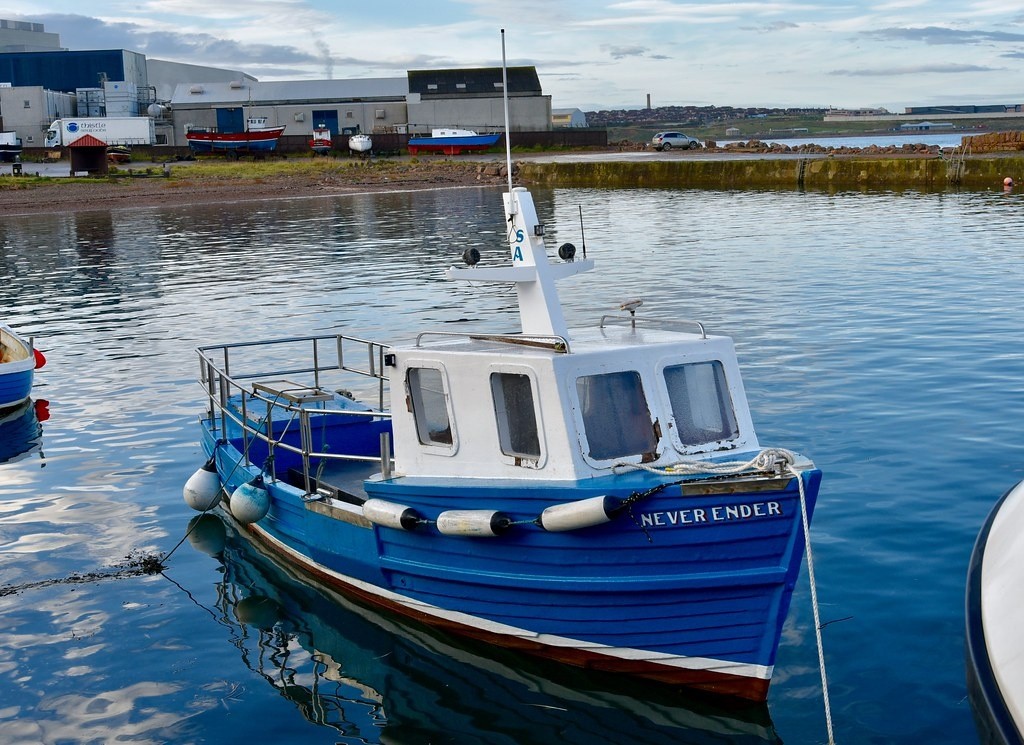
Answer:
[651,132,699,152]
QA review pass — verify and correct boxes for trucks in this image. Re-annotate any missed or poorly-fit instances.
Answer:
[45,116,157,149]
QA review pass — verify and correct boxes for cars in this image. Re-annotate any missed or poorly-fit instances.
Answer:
[0,400,48,470]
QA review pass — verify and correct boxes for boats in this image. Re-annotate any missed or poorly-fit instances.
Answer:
[180,25,827,714]
[308,138,333,153]
[407,127,503,154]
[0,321,46,410]
[348,133,373,151]
[183,86,287,151]
